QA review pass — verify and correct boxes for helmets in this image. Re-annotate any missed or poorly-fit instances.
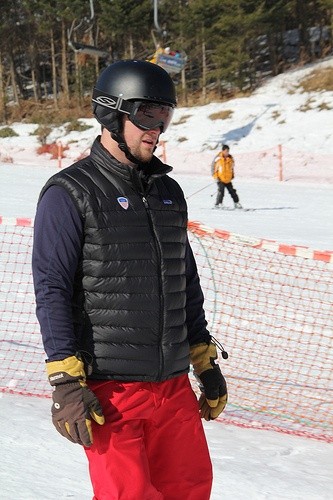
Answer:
[92,61,177,114]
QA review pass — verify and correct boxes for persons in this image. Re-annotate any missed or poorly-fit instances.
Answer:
[212,145,245,211]
[148,44,165,63]
[31,59,227,500]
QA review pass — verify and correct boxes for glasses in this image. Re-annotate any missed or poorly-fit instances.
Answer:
[92,88,173,133]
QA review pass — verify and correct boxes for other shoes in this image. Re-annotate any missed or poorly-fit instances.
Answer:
[235,203,242,209]
[216,204,220,208]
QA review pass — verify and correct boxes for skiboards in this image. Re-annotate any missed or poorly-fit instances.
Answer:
[211,208,256,212]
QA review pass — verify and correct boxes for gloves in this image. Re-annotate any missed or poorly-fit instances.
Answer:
[190,343,228,422]
[44,352,104,447]
